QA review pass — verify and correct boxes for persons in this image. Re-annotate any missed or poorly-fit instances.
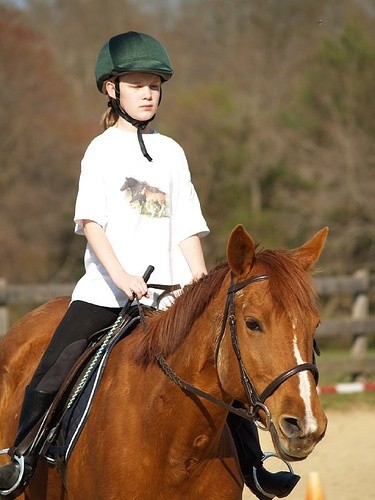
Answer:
[0,31,302,500]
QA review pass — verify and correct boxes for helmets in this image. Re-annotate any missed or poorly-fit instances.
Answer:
[94,31,174,95]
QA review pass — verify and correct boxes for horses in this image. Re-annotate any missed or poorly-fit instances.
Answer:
[0,222,332,500]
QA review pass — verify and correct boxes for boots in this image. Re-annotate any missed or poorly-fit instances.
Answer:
[0,383,58,500]
[228,401,301,500]
